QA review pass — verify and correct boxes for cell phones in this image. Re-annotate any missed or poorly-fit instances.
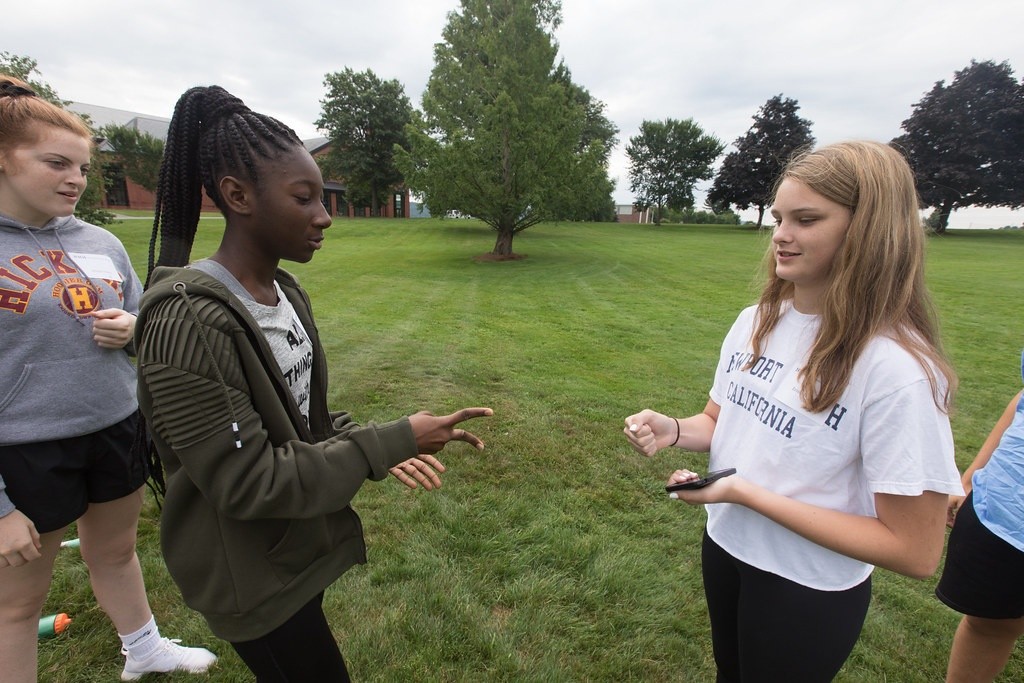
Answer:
[665,468,736,492]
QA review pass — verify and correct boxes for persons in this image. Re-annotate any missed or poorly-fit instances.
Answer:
[623,143,966,682]
[134,85,493,683]
[1,72,218,683]
[934,344,1024,683]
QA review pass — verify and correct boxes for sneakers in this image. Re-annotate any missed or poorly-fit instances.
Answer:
[121,637,218,682]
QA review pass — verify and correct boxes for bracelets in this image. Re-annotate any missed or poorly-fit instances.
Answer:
[670,418,680,446]
